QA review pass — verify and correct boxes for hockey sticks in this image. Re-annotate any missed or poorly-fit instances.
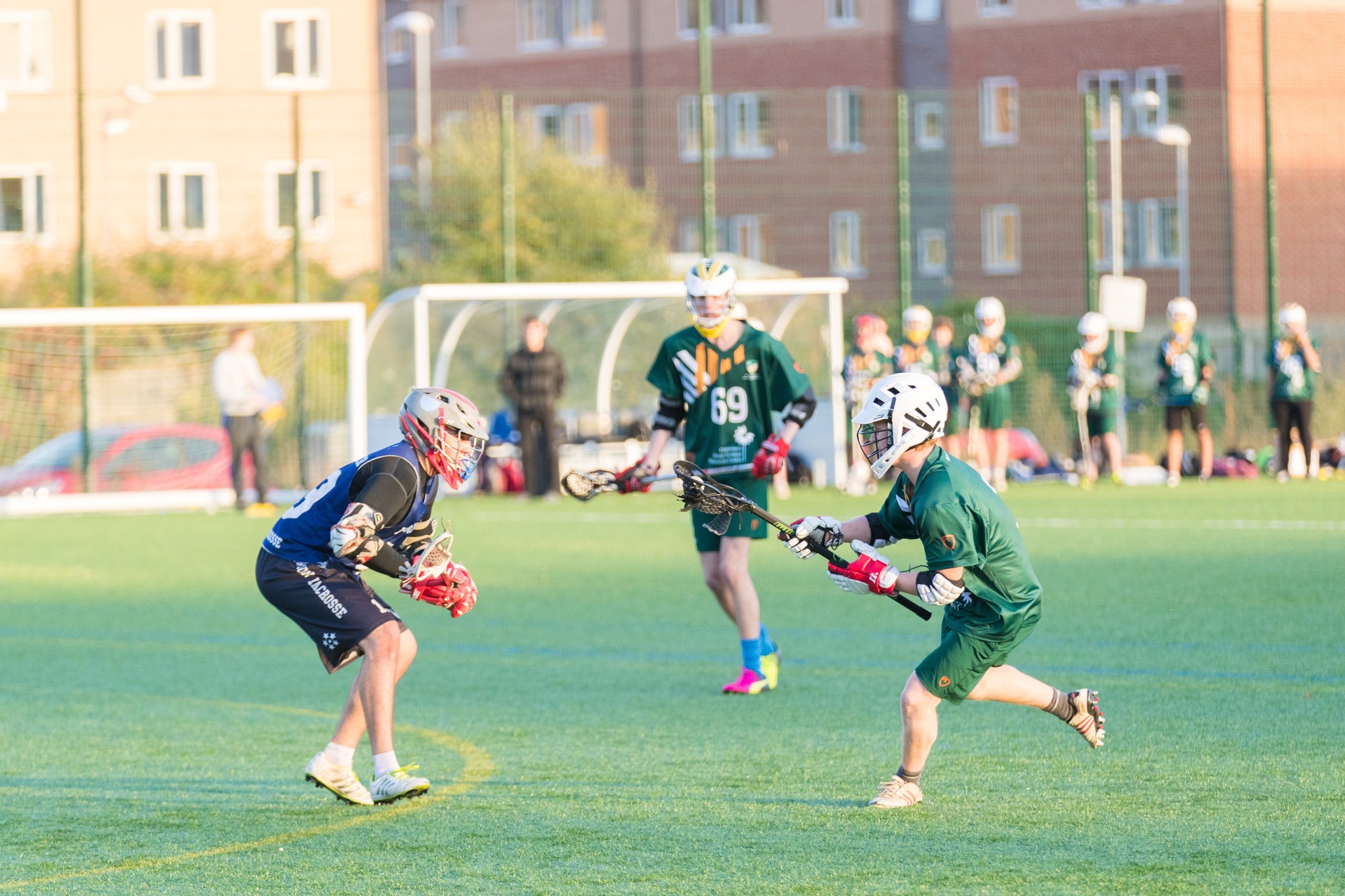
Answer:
[1076,411,1095,484]
[412,531,454,588]
[561,462,754,501]
[840,402,877,498]
[673,460,932,621]
[966,397,983,471]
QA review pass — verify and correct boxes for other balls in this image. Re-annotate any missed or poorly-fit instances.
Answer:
[686,476,705,491]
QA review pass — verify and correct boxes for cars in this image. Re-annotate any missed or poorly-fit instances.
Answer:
[0,420,255,498]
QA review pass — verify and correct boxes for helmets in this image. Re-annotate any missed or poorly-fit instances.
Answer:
[684,257,737,338]
[1076,310,1109,357]
[973,297,1006,340]
[851,373,948,480]
[1278,303,1307,326]
[902,305,933,346]
[1166,296,1197,325]
[398,386,489,491]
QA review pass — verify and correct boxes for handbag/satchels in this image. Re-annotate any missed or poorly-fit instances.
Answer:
[1212,454,1260,478]
[1160,451,1202,477]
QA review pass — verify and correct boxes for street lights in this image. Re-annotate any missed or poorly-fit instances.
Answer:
[385,9,433,206]
[1149,121,1194,299]
[1108,85,1165,455]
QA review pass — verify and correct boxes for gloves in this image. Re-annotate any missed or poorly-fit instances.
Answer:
[398,554,479,618]
[752,432,789,478]
[611,453,660,494]
[775,514,844,560]
[827,539,900,599]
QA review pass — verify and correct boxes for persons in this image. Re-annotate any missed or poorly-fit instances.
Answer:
[845,295,1021,490]
[616,259,818,695]
[1266,304,1321,478]
[1155,299,1216,482]
[778,375,1105,810]
[212,328,272,512]
[495,317,568,500]
[254,387,489,808]
[1064,311,1121,482]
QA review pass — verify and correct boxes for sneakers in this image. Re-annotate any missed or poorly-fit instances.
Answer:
[868,774,923,810]
[758,640,782,689]
[305,751,373,807]
[1067,688,1105,749]
[371,762,431,805]
[721,666,769,694]
